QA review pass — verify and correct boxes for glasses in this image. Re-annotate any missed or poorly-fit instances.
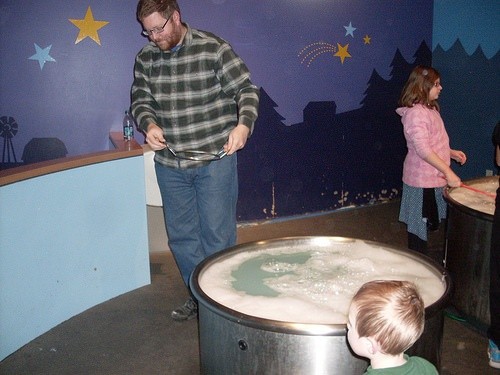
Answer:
[432,82,442,87]
[142,12,173,36]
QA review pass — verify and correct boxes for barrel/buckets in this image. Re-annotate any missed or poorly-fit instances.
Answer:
[189,234,450,375]
[441,173,500,335]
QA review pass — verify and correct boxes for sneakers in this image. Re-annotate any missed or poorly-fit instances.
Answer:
[171,295,199,320]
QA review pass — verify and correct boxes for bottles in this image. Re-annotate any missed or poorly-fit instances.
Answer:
[122,110,134,142]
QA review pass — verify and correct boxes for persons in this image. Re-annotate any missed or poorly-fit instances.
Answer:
[346,280,439,375]
[128,0,260,321]
[395,65,467,255]
[486,120,500,369]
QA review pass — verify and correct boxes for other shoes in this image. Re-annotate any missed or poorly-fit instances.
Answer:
[488,339,500,368]
